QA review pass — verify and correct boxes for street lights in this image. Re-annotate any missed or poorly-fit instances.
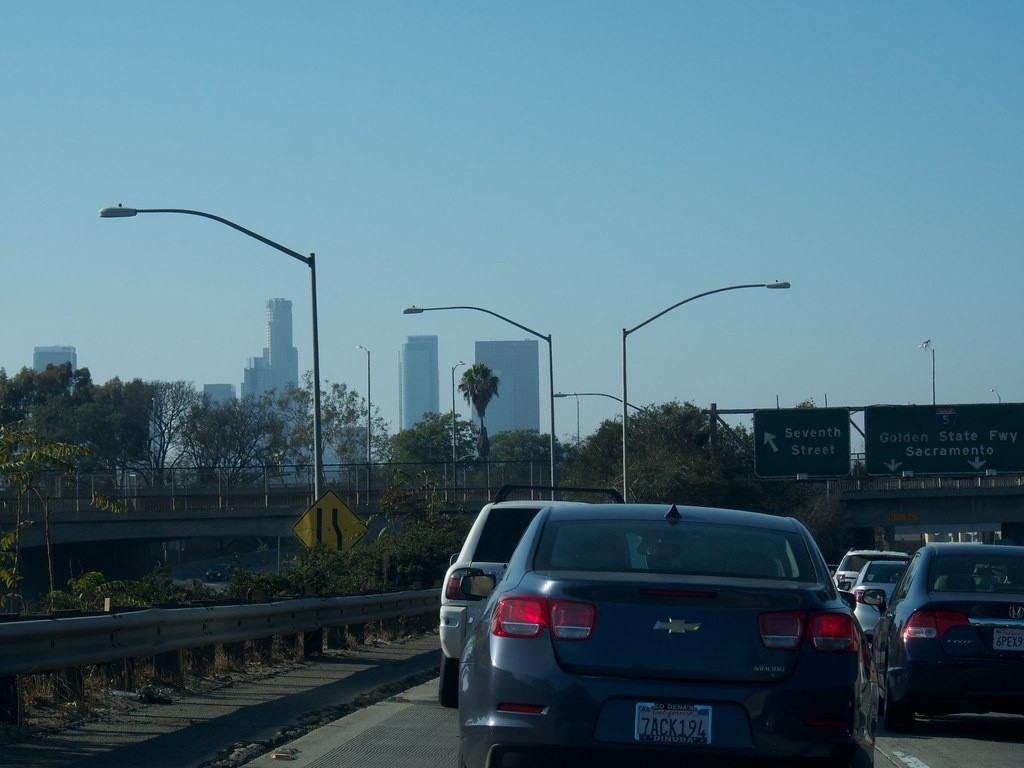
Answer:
[402,305,558,503]
[572,390,581,445]
[100,204,323,503]
[989,389,1001,405]
[920,338,939,402]
[355,344,370,506]
[452,363,465,506]
[623,281,791,504]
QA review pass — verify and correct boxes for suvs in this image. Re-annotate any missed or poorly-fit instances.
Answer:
[862,543,1024,736]
[851,559,912,645]
[832,548,909,607]
[454,501,878,768]
[437,501,589,709]
